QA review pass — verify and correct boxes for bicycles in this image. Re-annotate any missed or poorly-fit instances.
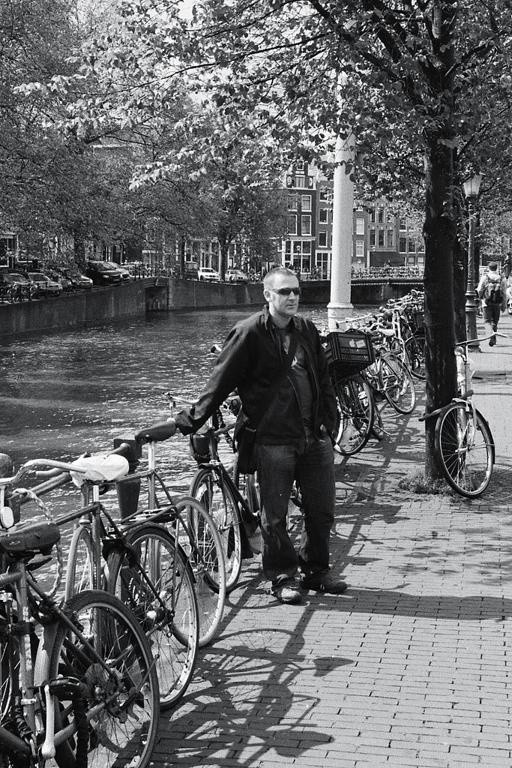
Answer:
[335,290,424,456]
[436,332,506,496]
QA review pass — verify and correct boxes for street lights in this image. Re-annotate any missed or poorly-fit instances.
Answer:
[463,168,483,350]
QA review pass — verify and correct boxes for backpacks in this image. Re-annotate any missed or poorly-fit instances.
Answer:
[485,274,503,307]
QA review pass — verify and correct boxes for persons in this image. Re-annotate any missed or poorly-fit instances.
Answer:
[384,258,392,274]
[475,262,506,346]
[246,263,321,282]
[166,263,348,605]
[500,251,512,280]
[500,249,506,266]
[141,263,159,278]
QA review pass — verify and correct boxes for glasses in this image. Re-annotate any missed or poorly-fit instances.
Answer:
[267,287,301,295]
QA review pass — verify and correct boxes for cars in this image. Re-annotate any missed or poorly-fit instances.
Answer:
[225,270,249,283]
[1,260,130,296]
[198,267,220,283]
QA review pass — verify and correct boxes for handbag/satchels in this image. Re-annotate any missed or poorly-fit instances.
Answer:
[233,404,257,475]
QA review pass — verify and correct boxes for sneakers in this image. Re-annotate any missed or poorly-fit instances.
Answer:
[489,335,496,346]
[271,579,302,604]
[299,568,346,593]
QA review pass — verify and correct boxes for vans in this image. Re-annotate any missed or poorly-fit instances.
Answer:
[185,260,199,278]
